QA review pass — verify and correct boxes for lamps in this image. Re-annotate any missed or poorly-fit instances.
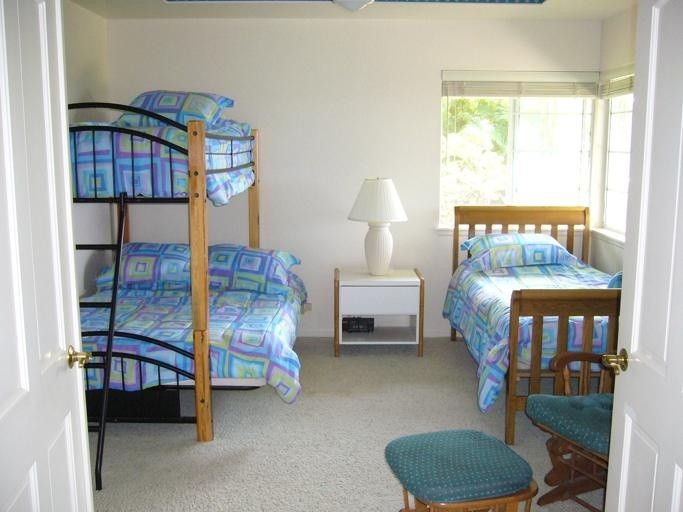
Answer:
[347,176,408,276]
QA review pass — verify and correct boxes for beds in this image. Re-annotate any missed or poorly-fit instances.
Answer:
[68,101,311,443]
[440,205,622,447]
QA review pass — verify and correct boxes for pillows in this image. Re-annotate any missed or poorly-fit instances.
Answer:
[461,232,588,273]
[96,239,191,290]
[209,243,302,293]
[114,89,235,131]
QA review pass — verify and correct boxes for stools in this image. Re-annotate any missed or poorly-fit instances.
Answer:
[384,430,538,511]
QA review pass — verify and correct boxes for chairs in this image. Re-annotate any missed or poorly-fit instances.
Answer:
[524,351,614,512]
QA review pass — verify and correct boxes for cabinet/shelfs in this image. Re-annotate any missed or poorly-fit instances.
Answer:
[333,268,424,357]
